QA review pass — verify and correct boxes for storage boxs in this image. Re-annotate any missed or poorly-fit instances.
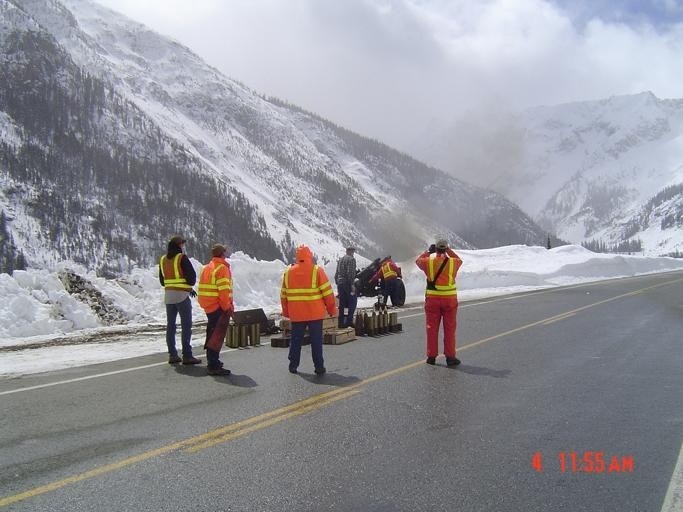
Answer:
[232,308,275,336]
[270,301,355,348]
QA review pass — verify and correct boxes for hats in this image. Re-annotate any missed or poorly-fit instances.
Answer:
[170,237,186,248]
[212,244,228,257]
[346,247,355,250]
[437,239,448,249]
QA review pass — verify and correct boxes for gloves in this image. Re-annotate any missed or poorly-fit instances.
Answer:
[429,244,436,253]
[189,290,197,298]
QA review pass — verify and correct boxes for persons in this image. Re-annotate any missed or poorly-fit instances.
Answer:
[334,247,357,328]
[159,234,201,364]
[280,243,337,374]
[368,256,400,306]
[415,239,463,366]
[198,243,234,376]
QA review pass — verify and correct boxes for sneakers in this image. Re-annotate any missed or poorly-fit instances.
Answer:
[168,351,201,365]
[446,359,461,366]
[207,368,231,376]
[314,368,327,376]
[288,367,297,373]
[427,358,435,364]
